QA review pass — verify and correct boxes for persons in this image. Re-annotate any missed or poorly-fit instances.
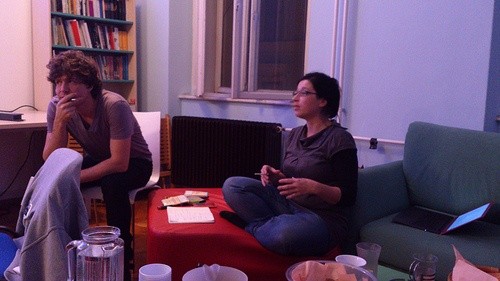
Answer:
[220,72,359,259]
[43,50,153,268]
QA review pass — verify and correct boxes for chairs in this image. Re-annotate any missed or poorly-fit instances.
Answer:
[63,111,172,248]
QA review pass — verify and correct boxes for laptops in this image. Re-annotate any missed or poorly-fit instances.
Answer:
[391,200,494,235]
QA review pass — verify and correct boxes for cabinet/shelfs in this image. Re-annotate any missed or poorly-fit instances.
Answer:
[32,0,138,114]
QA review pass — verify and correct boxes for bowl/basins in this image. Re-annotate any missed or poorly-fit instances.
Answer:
[285,260,379,281]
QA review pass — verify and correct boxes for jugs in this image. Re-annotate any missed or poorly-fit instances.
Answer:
[65,226,124,281]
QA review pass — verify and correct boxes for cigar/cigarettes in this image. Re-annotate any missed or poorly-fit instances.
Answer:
[59,98,77,101]
[255,173,269,176]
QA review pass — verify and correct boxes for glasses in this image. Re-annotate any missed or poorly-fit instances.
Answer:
[292,90,318,97]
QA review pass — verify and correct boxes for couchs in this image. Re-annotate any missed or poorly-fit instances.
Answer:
[352,121,500,281]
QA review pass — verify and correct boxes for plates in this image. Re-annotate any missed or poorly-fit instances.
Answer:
[448,264,500,281]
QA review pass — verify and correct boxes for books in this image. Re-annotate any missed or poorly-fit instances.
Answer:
[51,18,130,51]
[51,0,126,21]
[95,54,128,80]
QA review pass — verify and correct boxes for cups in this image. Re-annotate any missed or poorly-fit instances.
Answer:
[335,255,367,268]
[356,242,381,273]
[182,265,248,281]
[409,253,439,281]
[139,264,172,281]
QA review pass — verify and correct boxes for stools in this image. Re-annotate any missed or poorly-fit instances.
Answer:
[147,185,341,279]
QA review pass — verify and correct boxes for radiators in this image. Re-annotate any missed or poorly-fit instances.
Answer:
[171,115,283,189]
